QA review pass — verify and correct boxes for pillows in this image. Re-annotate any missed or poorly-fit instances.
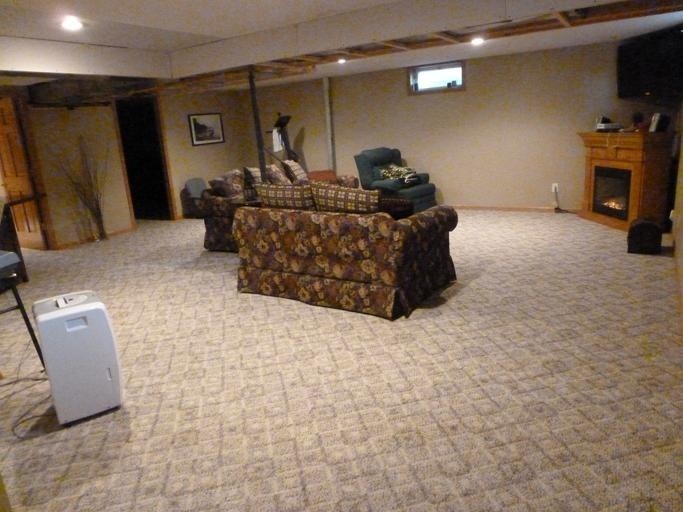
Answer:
[374,162,409,181]
[207,160,381,214]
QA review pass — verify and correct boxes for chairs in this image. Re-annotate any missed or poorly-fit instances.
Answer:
[186,178,209,218]
[352,147,437,213]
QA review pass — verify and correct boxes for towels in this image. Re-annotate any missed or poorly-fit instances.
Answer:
[272,128,285,153]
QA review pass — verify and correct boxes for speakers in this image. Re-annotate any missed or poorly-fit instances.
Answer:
[627,215,662,255]
[31,289,122,427]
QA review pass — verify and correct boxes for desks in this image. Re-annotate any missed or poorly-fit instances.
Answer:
[1,192,48,293]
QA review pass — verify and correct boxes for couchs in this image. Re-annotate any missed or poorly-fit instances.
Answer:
[200,159,359,252]
[232,179,459,322]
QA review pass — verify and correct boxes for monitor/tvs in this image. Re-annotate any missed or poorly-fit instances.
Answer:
[616,24,683,108]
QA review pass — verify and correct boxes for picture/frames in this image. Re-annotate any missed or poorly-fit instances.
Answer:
[188,112,226,146]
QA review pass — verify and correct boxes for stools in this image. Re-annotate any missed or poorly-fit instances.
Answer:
[0,249,45,380]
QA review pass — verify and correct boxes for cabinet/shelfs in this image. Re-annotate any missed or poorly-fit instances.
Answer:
[575,131,677,234]
